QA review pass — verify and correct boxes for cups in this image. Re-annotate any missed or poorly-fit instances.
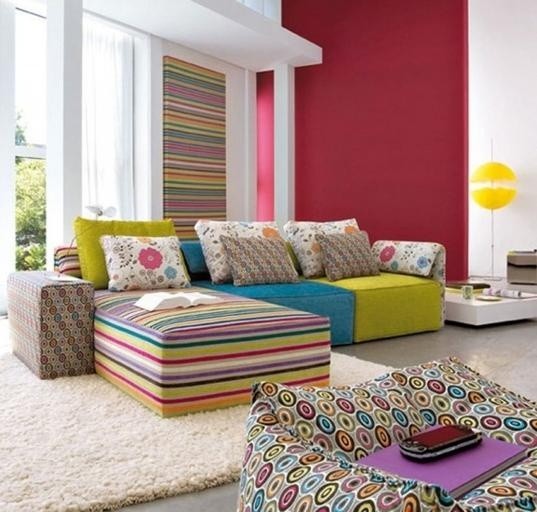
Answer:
[460,286,473,300]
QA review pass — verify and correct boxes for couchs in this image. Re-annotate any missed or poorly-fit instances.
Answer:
[54,239,446,420]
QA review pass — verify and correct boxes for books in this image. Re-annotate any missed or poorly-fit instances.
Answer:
[352,423,528,503]
[483,288,537,299]
[133,291,224,312]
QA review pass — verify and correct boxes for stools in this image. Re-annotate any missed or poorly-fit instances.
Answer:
[6,267,97,379]
[236,356,537,511]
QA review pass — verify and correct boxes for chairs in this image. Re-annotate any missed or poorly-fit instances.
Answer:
[506,247,537,284]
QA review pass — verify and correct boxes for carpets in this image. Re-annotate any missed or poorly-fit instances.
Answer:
[0,316,411,510]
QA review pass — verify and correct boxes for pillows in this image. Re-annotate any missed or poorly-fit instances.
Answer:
[214,232,302,290]
[283,215,362,277]
[98,233,193,296]
[194,214,299,282]
[74,214,196,291]
[315,230,380,281]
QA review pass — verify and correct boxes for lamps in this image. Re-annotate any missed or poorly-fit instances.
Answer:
[469,136,517,280]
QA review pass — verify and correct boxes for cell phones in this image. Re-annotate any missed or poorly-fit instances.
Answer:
[398,424,482,462]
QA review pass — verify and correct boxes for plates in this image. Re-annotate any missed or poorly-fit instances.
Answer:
[476,296,499,301]
